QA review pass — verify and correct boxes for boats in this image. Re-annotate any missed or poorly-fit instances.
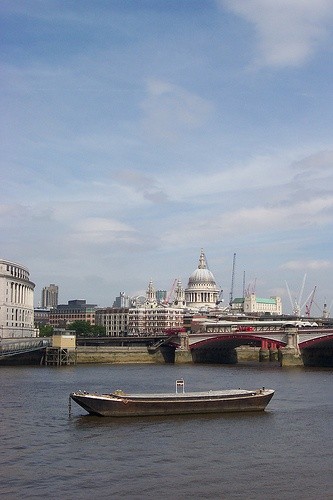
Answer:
[67,381,276,418]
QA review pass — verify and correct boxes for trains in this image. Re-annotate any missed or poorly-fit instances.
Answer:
[204,321,319,334]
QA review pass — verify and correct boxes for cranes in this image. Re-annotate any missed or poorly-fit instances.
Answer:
[306,289,320,318]
[162,278,176,306]
[217,253,259,313]
[286,275,315,317]
